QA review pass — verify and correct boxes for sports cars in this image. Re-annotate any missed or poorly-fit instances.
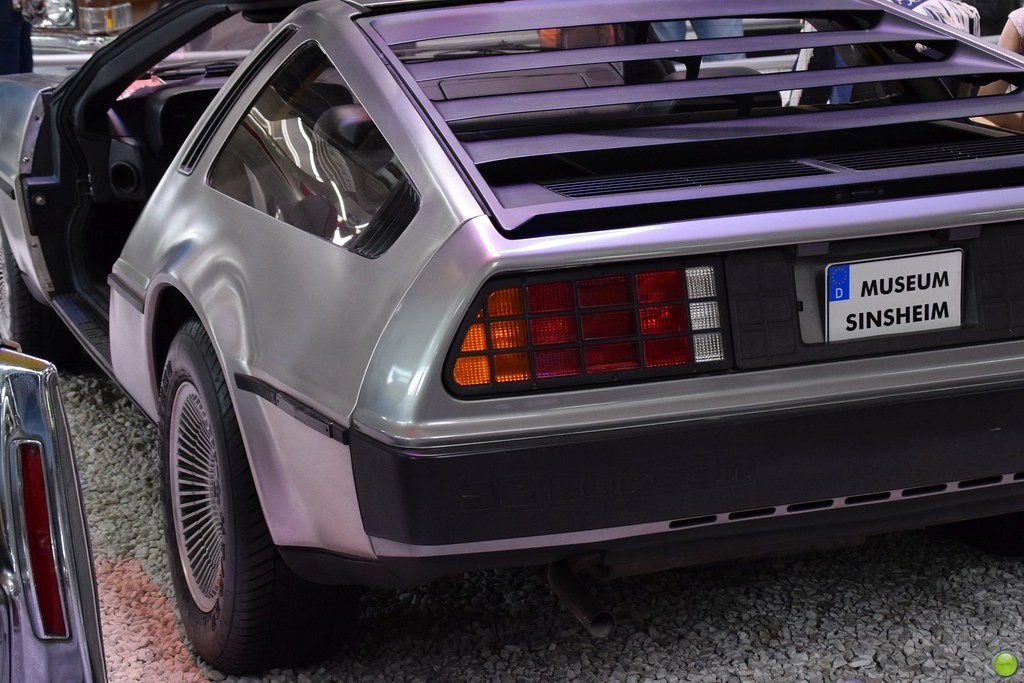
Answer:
[0,0,1024,678]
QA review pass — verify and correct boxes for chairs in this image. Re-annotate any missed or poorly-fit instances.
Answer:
[290,84,354,129]
[654,67,784,117]
